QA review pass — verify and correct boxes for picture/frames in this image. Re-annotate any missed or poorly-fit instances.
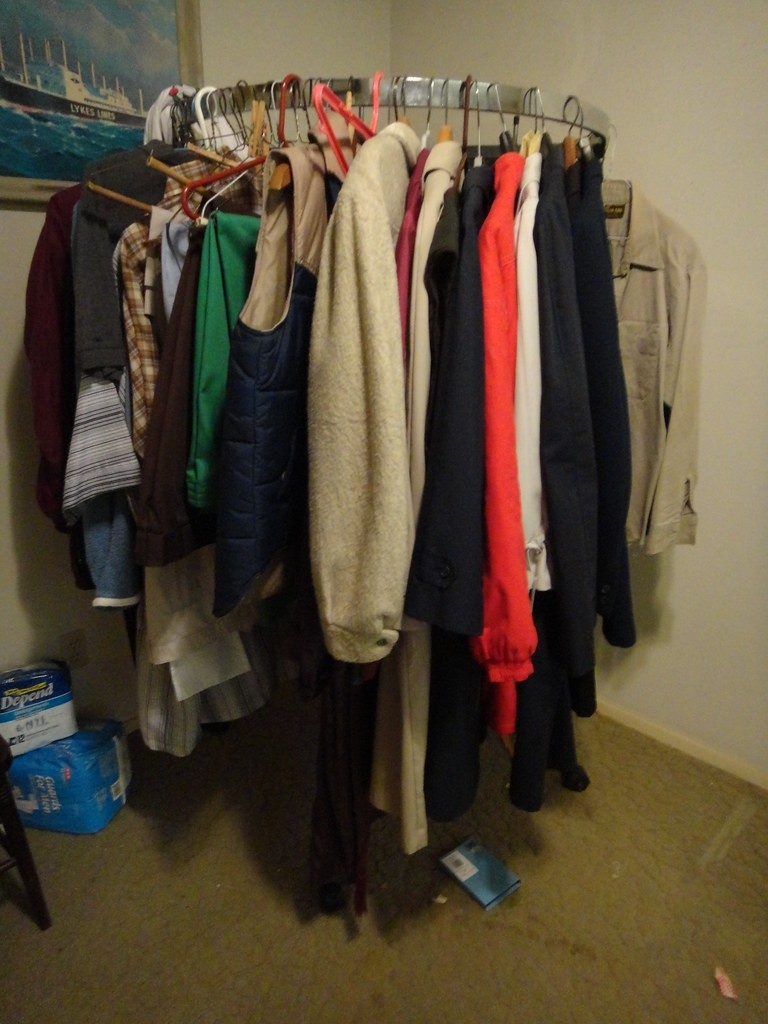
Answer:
[0,0,206,204]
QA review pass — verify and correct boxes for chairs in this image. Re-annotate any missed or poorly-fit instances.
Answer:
[0,736,52,931]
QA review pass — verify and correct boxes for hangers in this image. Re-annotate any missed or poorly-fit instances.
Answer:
[143,72,632,228]
